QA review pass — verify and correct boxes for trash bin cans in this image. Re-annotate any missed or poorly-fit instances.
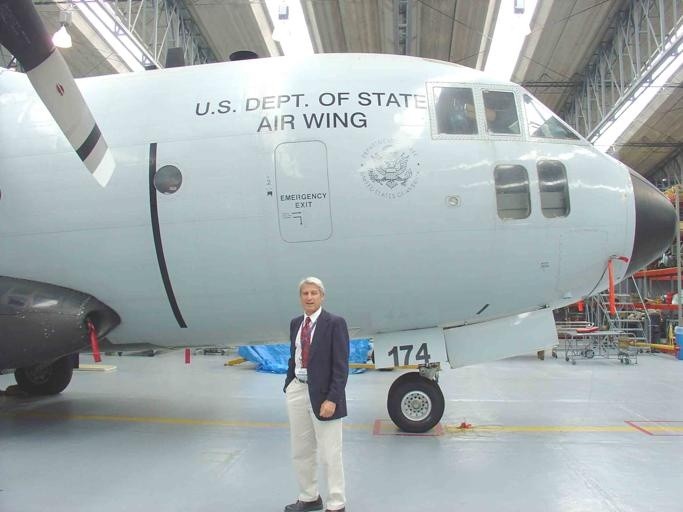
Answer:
[674,325,683,360]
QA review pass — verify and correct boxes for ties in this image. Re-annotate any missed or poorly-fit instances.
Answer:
[299,316,312,370]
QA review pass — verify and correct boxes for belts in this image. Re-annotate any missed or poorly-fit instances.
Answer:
[295,377,309,385]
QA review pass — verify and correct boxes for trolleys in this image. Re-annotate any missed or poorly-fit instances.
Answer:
[552,320,595,359]
[563,329,641,366]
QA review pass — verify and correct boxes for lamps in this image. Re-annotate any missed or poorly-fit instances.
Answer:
[51,3,73,49]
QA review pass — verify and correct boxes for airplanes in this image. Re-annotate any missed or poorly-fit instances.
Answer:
[0,0,683,438]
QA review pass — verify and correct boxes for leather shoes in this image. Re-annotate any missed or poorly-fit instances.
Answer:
[282,495,324,512]
[325,507,345,512]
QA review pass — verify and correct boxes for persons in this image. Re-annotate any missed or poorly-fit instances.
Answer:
[283,277,349,512]
